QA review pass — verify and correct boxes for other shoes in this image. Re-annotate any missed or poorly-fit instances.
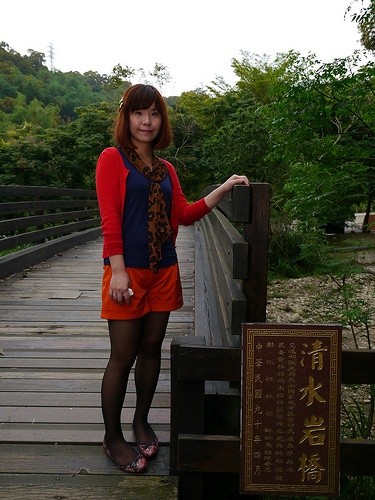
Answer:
[103,435,148,473]
[132,421,159,457]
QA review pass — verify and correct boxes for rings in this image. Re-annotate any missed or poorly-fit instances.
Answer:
[112,294,116,295]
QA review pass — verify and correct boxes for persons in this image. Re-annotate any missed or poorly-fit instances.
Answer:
[96,84,250,473]
